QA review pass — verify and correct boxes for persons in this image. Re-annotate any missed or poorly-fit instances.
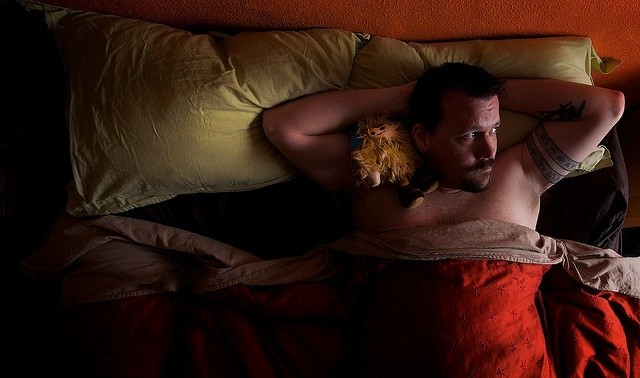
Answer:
[262,62,625,378]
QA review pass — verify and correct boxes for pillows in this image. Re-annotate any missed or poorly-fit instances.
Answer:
[36,3,370,218]
[349,31,627,181]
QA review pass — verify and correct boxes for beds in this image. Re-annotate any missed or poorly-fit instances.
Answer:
[10,0,640,378]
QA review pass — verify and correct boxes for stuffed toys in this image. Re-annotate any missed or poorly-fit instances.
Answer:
[351,109,440,209]
